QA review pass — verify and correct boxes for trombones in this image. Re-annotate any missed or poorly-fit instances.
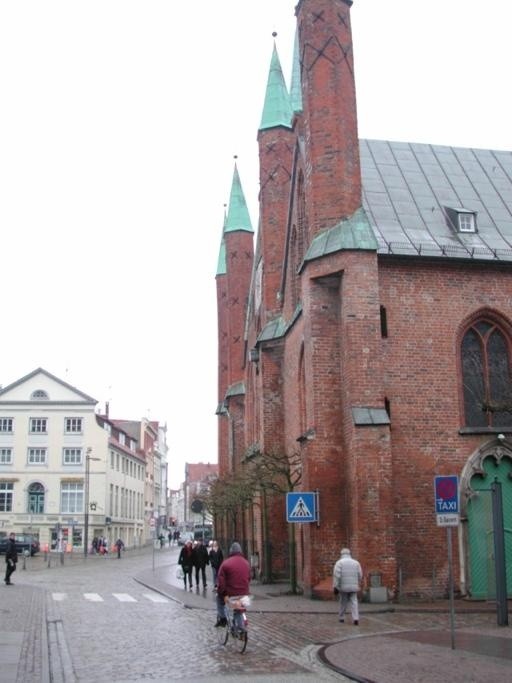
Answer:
[287,492,315,523]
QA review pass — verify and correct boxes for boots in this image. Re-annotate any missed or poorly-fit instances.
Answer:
[0,532,40,557]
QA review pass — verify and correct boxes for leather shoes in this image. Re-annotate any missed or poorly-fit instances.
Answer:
[333,587,339,594]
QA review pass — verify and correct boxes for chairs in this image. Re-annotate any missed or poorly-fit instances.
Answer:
[5,578,14,585]
[214,617,228,627]
[339,619,344,623]
[184,584,218,589]
[353,620,359,625]
[232,631,245,640]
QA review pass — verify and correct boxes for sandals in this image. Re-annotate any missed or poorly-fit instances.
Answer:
[193,521,212,540]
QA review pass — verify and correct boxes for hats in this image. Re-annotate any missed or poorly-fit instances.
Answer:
[178,531,193,545]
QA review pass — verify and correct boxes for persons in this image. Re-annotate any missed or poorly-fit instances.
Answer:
[92,535,124,558]
[3,533,18,585]
[158,529,223,592]
[214,541,251,637]
[333,547,363,625]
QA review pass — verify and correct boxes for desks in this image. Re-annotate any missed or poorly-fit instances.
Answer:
[82,449,102,558]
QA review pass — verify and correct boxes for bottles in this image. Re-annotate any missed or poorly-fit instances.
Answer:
[229,541,242,553]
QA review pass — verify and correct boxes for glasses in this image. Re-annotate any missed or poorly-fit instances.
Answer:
[497,432,506,445]
